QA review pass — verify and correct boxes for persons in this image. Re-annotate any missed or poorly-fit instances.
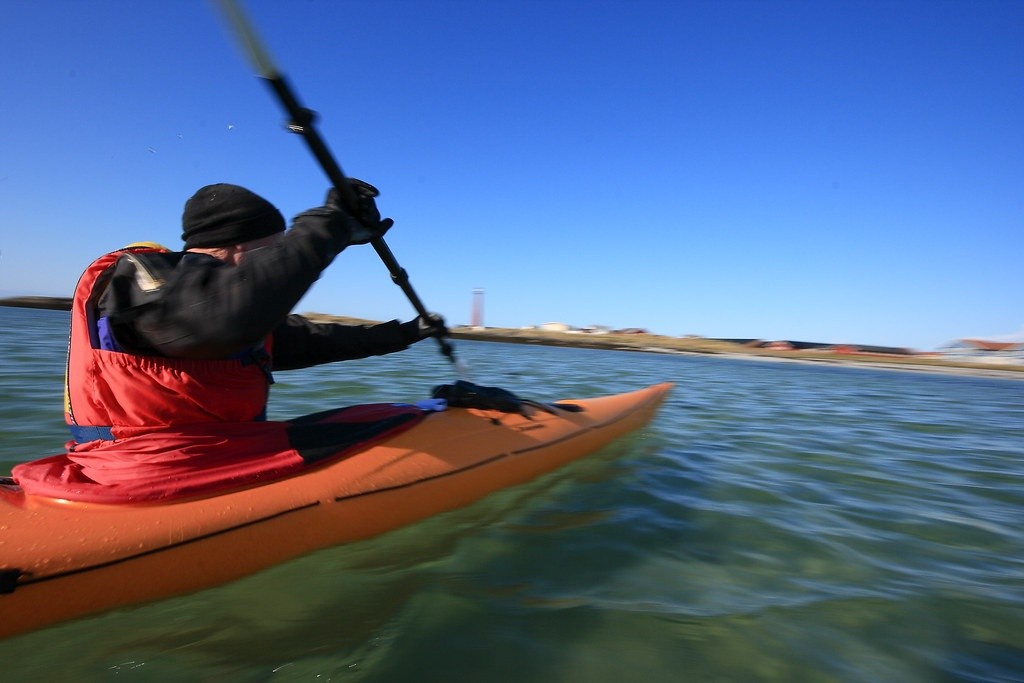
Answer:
[63,177,451,485]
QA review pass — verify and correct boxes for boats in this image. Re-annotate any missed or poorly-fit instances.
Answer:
[0,367,674,644]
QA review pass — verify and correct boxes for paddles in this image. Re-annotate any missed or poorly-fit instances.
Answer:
[209,0,476,386]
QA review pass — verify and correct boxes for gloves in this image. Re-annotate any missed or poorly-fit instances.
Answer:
[401,312,447,346]
[325,177,395,246]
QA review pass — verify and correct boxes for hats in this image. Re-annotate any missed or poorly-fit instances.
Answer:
[181,182,287,251]
